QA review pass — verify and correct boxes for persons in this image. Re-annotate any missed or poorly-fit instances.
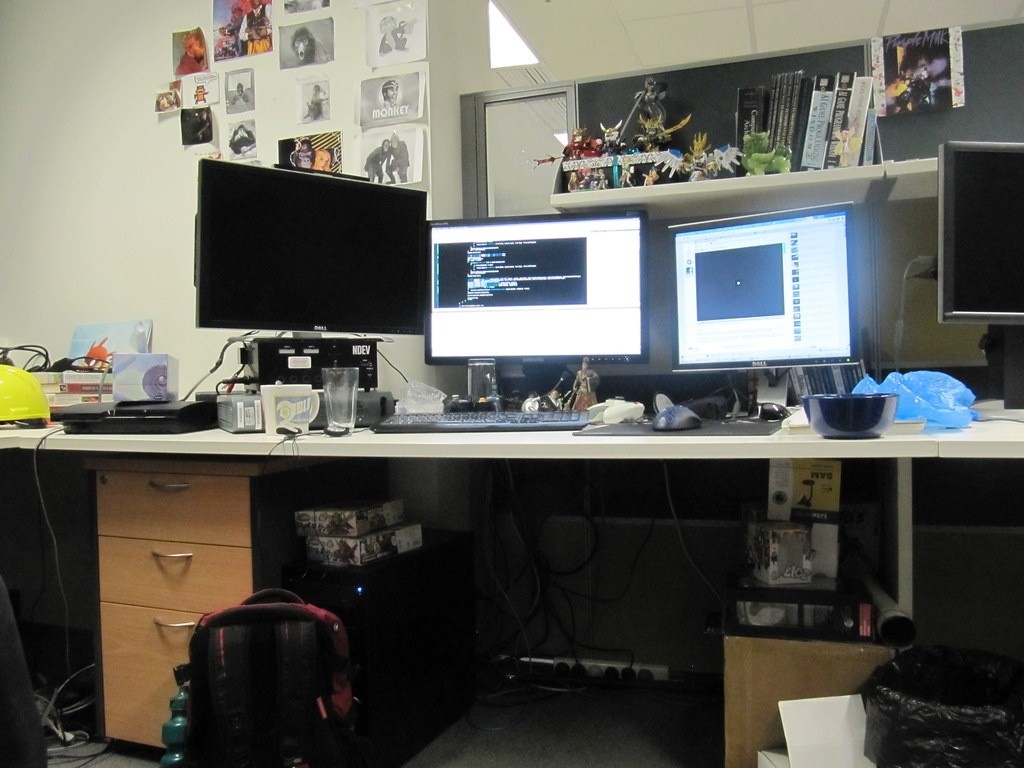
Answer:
[382,80,399,110]
[309,85,326,121]
[313,149,332,172]
[234,83,248,104]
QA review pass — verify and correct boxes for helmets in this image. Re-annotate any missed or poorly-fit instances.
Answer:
[0,364,50,429]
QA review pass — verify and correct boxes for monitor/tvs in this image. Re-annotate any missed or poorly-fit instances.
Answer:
[666,200,862,422]
[423,210,650,412]
[193,158,427,339]
[937,141,1024,425]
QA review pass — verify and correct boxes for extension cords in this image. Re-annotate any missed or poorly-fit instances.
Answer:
[551,658,670,684]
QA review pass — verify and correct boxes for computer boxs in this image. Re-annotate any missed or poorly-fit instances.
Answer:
[215,389,396,434]
[282,529,478,768]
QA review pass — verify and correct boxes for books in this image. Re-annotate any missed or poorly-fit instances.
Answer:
[734,69,877,171]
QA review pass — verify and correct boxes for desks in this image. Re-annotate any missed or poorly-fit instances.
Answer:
[0,408,1024,741]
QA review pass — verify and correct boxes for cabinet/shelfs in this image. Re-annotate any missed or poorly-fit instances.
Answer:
[458,16,1024,379]
[82,453,343,752]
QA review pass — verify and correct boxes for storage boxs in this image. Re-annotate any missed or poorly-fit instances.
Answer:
[295,493,423,566]
[113,353,179,403]
[29,371,120,412]
[723,457,898,768]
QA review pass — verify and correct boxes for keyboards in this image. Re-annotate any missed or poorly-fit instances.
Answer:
[371,412,590,434]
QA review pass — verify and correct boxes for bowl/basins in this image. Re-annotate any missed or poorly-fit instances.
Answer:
[802,394,900,438]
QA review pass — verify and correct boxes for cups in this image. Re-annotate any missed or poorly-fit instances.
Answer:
[468,358,497,398]
[322,367,359,436]
[260,385,319,434]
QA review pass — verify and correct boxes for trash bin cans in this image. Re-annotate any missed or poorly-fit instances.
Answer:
[864,643,1024,768]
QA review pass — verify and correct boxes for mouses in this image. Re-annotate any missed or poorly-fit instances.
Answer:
[652,405,702,431]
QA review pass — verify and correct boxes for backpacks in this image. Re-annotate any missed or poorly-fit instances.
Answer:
[188,587,356,768]
